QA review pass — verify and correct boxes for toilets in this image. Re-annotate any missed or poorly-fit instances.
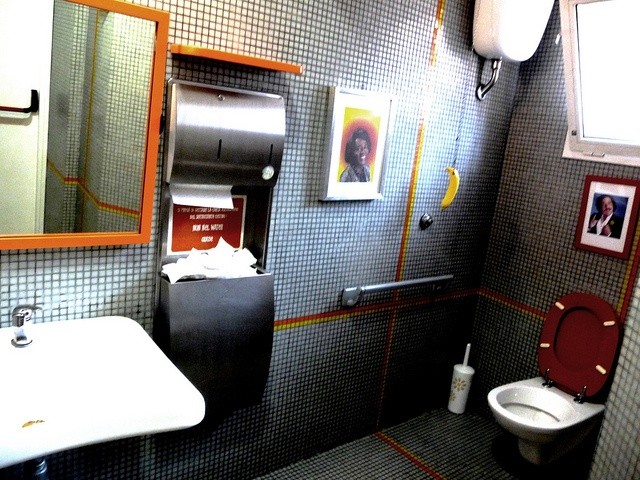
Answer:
[487,291,620,467]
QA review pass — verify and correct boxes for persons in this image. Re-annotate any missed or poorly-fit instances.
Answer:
[588,195,623,239]
[341,129,372,182]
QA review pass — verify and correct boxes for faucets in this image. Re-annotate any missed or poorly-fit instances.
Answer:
[11,305,42,344]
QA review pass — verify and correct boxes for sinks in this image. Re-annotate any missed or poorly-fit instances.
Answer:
[0,316,207,469]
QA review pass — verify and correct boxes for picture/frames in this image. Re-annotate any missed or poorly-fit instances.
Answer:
[318,85,398,202]
[574,175,640,260]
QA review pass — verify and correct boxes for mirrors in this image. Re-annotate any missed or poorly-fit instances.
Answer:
[0,1,169,250]
